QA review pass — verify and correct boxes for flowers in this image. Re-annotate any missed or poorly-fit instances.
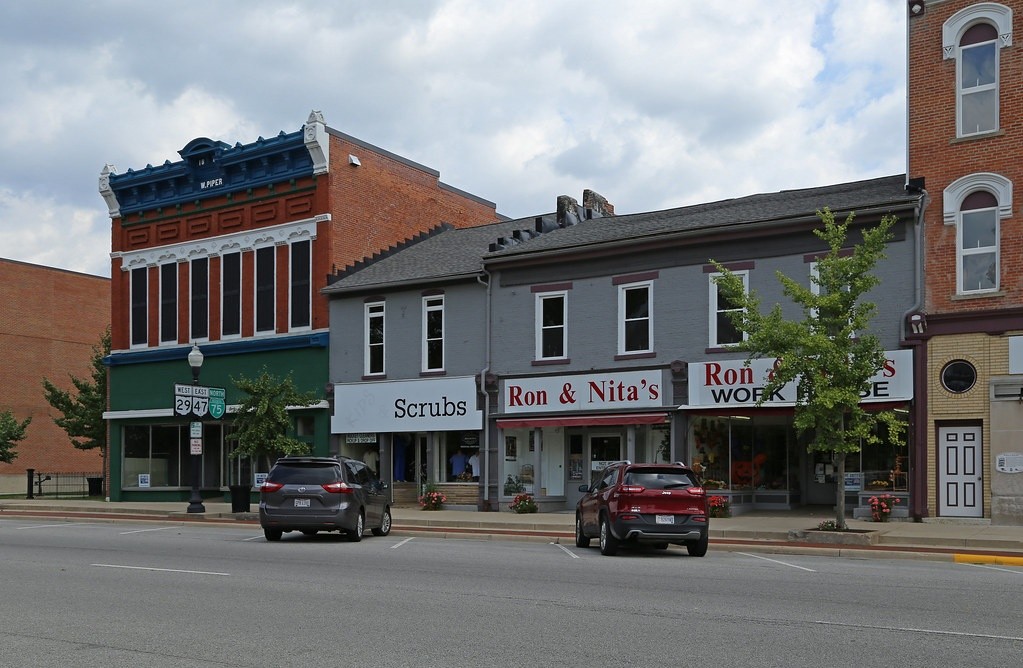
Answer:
[508,495,539,512]
[707,496,732,518]
[417,484,447,511]
[868,494,901,520]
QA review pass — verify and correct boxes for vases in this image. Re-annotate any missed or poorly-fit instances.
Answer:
[871,497,896,522]
[515,507,538,514]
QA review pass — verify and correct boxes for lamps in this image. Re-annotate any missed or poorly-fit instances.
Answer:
[349,154,362,166]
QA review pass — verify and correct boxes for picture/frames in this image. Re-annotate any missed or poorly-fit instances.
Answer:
[528,430,543,451]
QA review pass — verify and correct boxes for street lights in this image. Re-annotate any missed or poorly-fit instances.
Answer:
[187,340,206,513]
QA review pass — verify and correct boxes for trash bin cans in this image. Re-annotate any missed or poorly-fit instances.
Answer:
[86,478,103,496]
[230,484,253,513]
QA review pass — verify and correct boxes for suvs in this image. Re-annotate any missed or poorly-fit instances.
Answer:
[259,453,393,539]
[576,459,710,558]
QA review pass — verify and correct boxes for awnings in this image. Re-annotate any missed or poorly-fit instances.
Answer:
[494,412,672,429]
[677,397,913,416]
[102,400,331,421]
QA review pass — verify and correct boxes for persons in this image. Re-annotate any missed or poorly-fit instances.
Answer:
[450,449,469,477]
[394,433,408,483]
[363,447,379,476]
[469,448,480,481]
